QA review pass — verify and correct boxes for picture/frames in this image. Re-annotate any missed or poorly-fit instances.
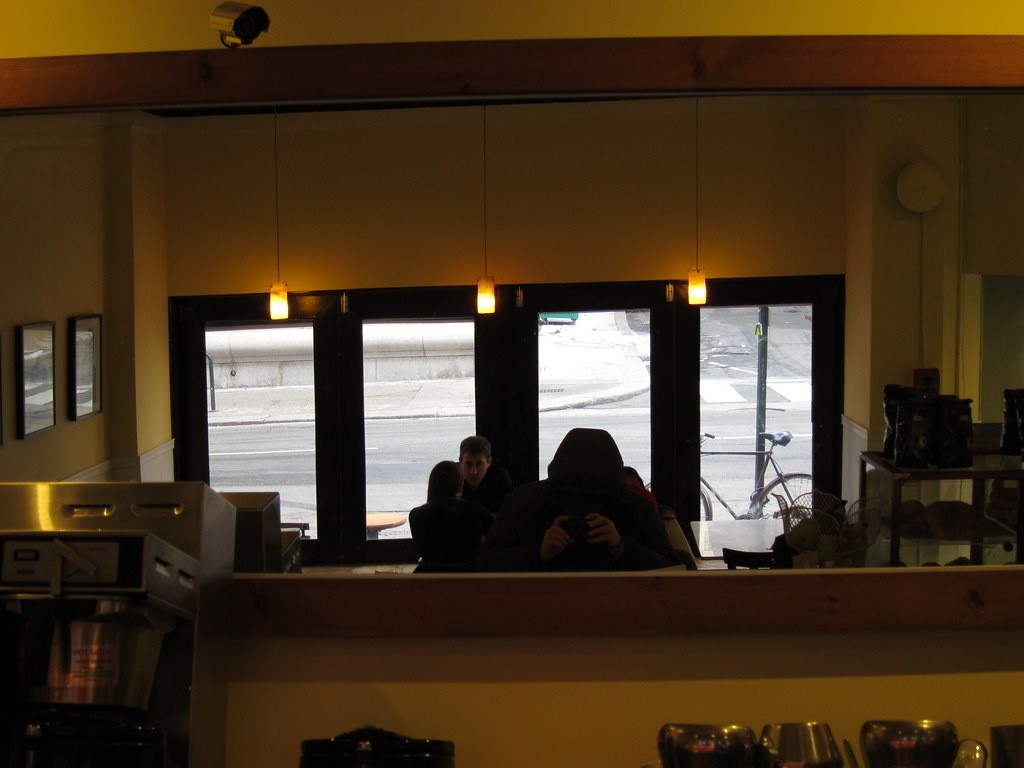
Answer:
[69,314,103,422]
[15,321,57,440]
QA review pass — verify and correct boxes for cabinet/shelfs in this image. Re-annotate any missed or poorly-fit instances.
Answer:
[859,451,1024,564]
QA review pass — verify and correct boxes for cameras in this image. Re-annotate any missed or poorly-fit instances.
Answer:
[561,518,591,541]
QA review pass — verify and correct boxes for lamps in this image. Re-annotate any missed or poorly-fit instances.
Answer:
[688,96,706,305]
[269,110,288,320]
[477,103,495,314]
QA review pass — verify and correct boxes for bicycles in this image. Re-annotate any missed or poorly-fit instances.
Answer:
[644,432,813,520]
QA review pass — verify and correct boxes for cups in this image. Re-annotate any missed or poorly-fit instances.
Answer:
[858,719,988,768]
[759,722,844,768]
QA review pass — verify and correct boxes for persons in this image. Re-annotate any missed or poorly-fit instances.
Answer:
[409,461,491,574]
[622,467,699,561]
[477,427,683,573]
[456,437,517,544]
